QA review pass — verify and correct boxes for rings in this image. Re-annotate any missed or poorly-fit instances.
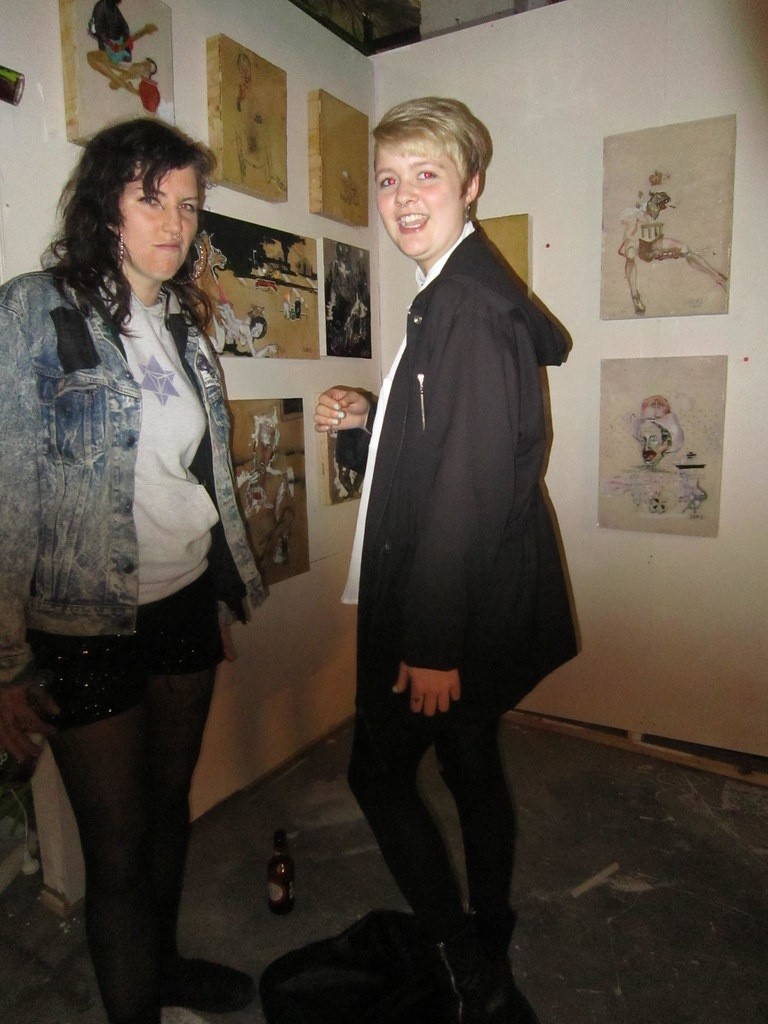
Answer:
[411,697,423,702]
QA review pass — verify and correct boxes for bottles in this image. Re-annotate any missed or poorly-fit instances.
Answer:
[0,680,49,792]
[267,830,295,916]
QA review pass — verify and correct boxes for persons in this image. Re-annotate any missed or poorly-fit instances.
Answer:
[0,117,272,1023]
[312,96,587,1024]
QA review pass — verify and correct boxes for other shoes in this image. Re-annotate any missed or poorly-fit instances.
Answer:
[162,955,255,1013]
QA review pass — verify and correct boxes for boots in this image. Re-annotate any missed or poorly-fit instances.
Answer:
[428,900,540,1024]
